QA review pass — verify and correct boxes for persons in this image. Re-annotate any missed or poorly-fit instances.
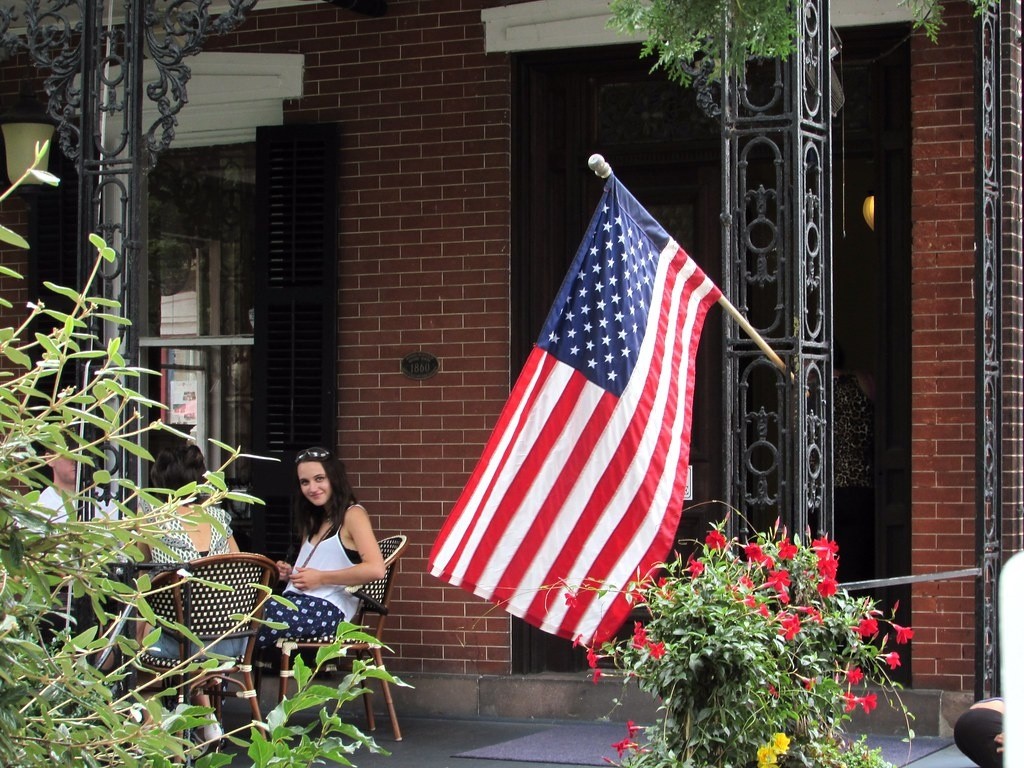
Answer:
[27,426,387,755]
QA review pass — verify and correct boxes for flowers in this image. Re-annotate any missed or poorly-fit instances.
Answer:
[565,532,915,768]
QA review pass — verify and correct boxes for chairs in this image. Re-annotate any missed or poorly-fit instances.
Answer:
[253,535,410,742]
[122,552,280,763]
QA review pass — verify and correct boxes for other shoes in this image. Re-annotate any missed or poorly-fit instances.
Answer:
[192,713,222,761]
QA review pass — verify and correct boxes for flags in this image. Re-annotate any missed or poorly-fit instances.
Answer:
[430,176,723,650]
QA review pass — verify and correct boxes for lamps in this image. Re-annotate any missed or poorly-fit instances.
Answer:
[0,65,56,211]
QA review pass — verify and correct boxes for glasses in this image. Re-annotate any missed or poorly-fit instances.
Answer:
[295,447,329,462]
